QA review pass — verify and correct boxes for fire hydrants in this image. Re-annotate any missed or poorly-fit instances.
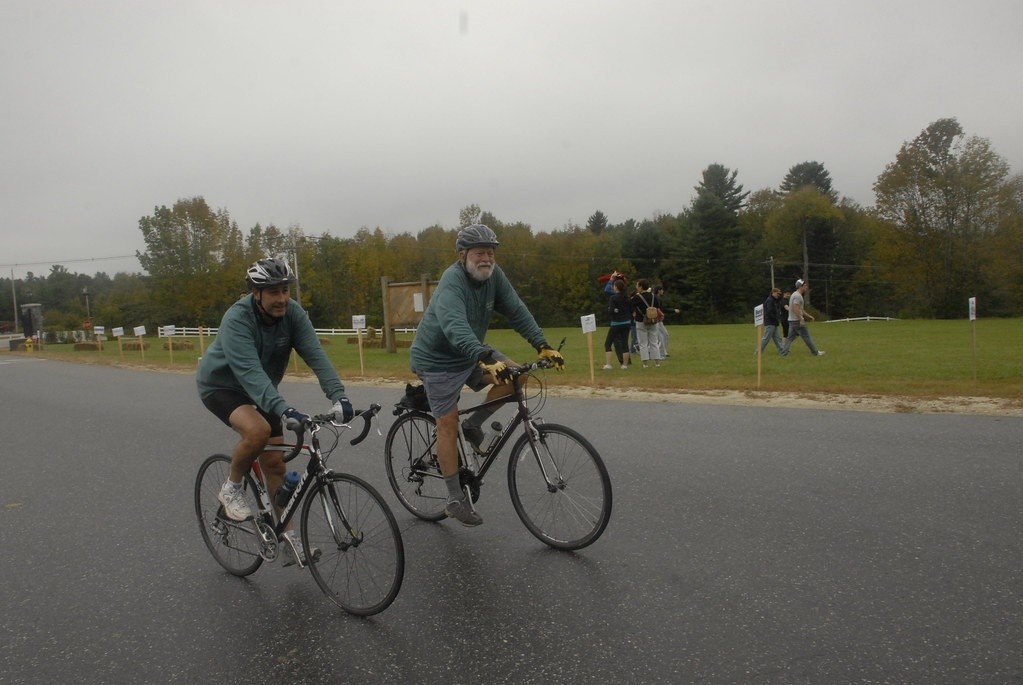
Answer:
[25,338,33,353]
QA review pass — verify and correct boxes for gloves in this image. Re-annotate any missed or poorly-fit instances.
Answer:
[478,350,514,387]
[327,397,355,424]
[282,408,313,432]
[538,343,566,372]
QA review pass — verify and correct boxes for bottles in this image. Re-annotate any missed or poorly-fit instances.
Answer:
[273,472,300,509]
[478,421,503,452]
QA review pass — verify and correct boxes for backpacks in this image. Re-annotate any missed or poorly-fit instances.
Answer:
[635,292,659,326]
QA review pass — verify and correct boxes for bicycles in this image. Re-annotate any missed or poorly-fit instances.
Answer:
[385,337,613,551]
[194,404,405,617]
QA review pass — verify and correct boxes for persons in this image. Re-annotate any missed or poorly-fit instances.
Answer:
[754,288,791,358]
[604,271,632,366]
[614,278,661,368]
[782,288,800,341]
[408,224,568,528]
[654,286,670,360]
[784,278,827,356]
[602,280,635,370]
[196,257,358,568]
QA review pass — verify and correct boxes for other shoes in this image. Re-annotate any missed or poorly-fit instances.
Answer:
[655,363,660,368]
[621,365,628,370]
[603,364,613,369]
[816,350,825,357]
[642,364,649,368]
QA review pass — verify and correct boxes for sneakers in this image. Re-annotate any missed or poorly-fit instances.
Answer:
[462,418,490,457]
[281,536,321,567]
[444,492,484,527]
[217,482,253,522]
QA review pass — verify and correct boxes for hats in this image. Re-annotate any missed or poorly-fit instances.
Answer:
[795,279,804,288]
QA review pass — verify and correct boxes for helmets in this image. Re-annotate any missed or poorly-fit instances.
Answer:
[245,257,297,289]
[455,224,500,251]
